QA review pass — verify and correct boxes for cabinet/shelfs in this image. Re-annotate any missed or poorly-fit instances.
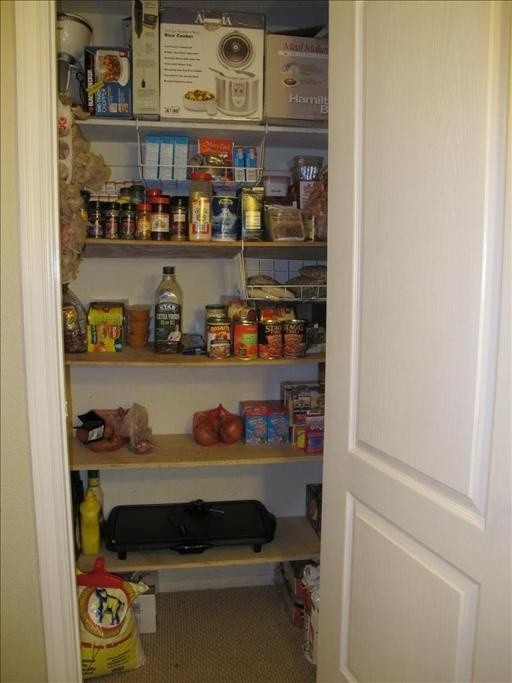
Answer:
[46,2,334,574]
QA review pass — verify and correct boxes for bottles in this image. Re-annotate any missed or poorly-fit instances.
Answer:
[146,188,162,205]
[212,196,240,241]
[144,136,160,179]
[156,266,182,353]
[300,180,318,208]
[88,201,103,239]
[130,184,145,204]
[85,470,103,523]
[240,400,268,448]
[159,136,174,180]
[267,400,290,447]
[244,148,258,182]
[120,203,137,239]
[232,146,245,181]
[189,173,213,242]
[103,202,120,239]
[239,187,264,239]
[118,188,131,201]
[171,196,189,241]
[265,202,304,242]
[149,197,171,241]
[174,136,190,180]
[136,202,152,240]
[79,489,101,556]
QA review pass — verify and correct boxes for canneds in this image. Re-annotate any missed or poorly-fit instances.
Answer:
[206,305,226,318]
[233,316,258,360]
[259,318,282,360]
[206,318,231,359]
[282,319,306,359]
